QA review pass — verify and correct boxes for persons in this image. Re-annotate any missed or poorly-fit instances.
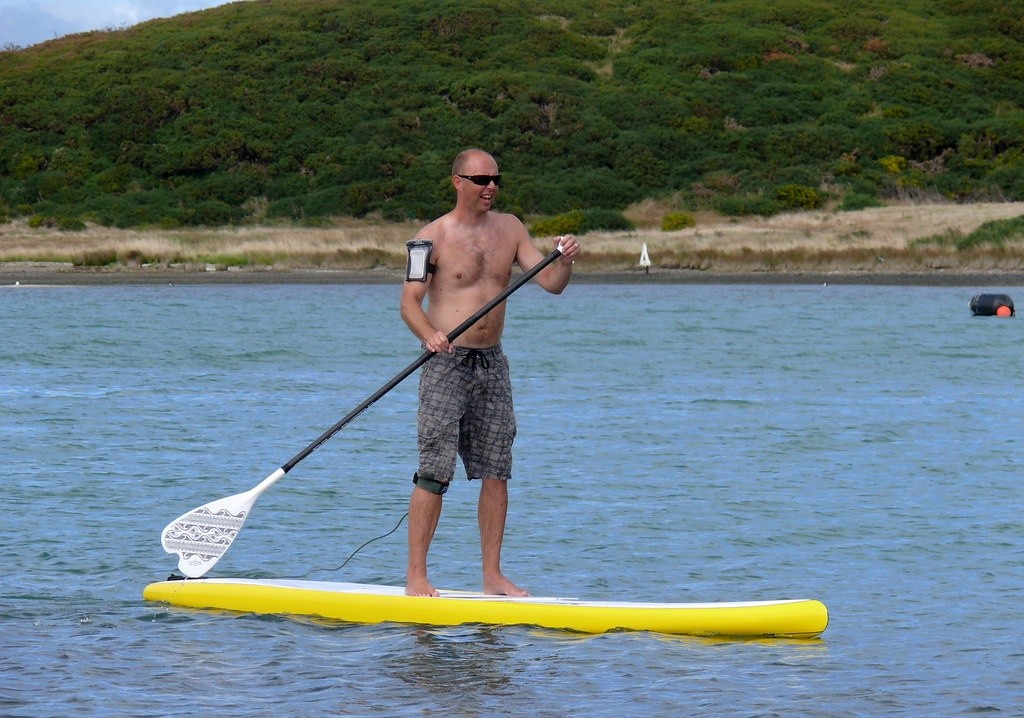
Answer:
[402,148,582,600]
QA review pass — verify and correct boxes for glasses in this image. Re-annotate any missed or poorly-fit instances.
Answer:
[458,174,502,185]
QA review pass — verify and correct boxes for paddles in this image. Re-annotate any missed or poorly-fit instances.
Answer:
[157,235,565,579]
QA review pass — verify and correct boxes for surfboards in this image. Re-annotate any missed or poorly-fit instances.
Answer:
[143,578,830,640]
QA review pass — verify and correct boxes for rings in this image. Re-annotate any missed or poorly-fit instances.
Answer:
[575,244,578,247]
